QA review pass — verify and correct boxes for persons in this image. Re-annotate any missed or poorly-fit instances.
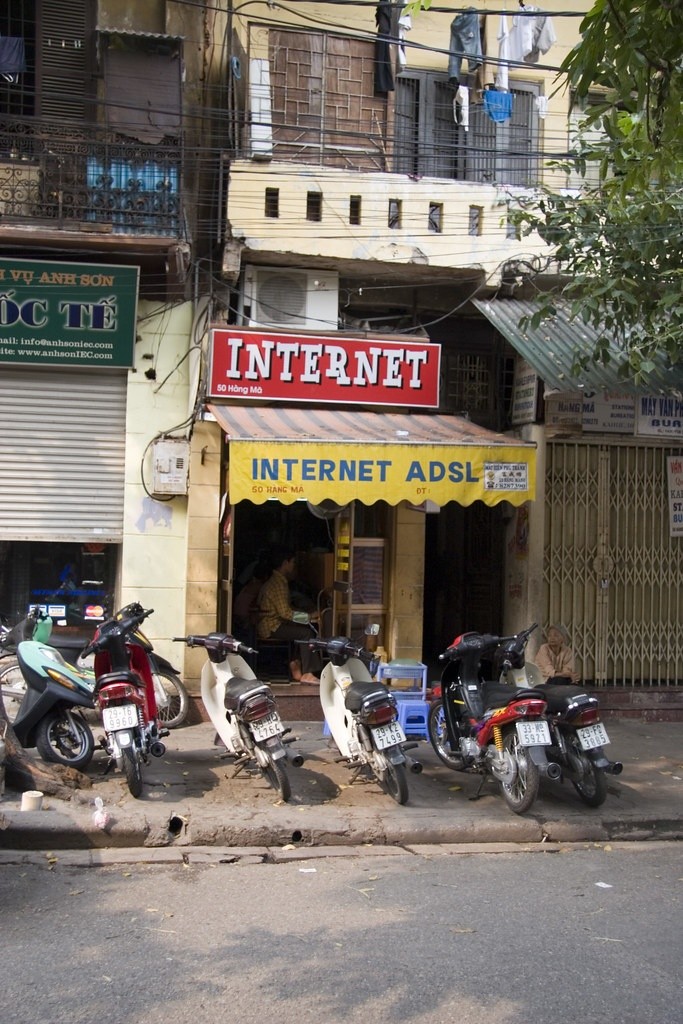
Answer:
[233,546,321,684]
[58,553,79,603]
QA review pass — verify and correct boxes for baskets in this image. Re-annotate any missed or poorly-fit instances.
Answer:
[352,650,381,678]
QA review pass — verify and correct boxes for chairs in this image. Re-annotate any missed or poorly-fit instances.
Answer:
[246,603,295,682]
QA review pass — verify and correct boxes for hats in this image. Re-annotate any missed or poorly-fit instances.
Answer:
[546,624,566,641]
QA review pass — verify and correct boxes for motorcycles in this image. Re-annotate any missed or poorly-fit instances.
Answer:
[291,610,424,804]
[426,622,624,815]
[0,601,190,800]
[171,632,305,801]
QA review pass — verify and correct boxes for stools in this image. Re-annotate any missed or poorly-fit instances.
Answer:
[396,699,431,742]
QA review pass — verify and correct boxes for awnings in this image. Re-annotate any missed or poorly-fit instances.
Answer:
[208,402,537,506]
